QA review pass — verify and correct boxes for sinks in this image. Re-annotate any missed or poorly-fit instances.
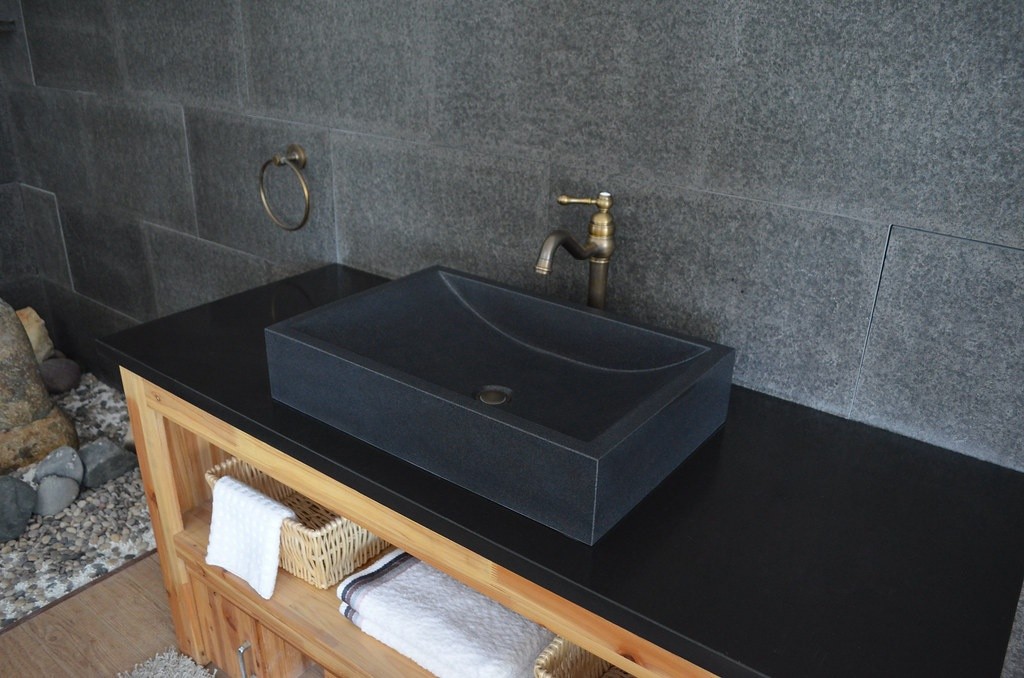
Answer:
[261,262,739,549]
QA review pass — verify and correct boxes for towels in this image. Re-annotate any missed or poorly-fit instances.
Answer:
[336,546,558,678]
[204,474,299,601]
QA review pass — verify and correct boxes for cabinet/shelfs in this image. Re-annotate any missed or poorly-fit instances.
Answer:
[95,262,1024,678]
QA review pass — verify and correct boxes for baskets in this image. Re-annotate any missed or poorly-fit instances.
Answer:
[534,634,637,678]
[204,456,392,590]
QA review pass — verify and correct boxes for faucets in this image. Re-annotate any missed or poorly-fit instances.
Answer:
[533,189,619,311]
